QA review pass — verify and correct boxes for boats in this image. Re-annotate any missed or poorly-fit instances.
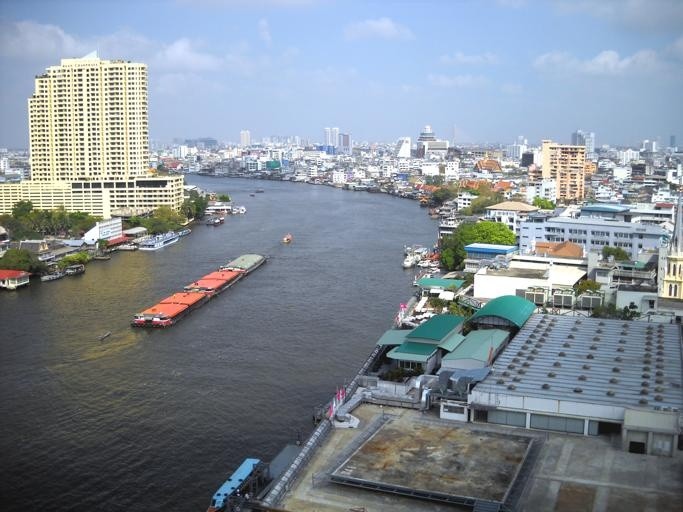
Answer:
[283,233,291,243]
[231,205,246,215]
[206,215,224,226]
[117,228,192,252]
[65,264,85,276]
[40,272,62,282]
[207,457,262,512]
[401,245,439,279]
